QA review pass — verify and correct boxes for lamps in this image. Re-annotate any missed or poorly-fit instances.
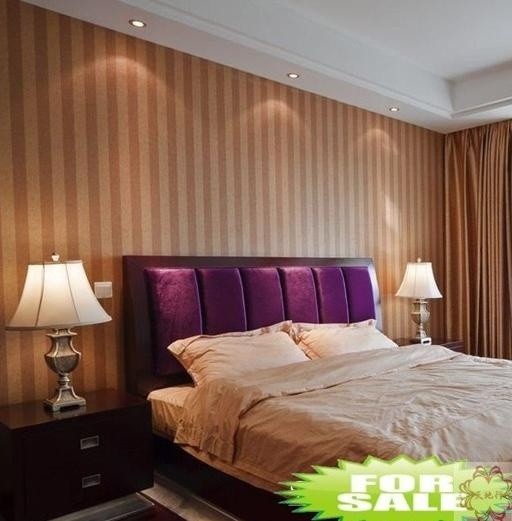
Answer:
[5,251,112,413]
[393,257,443,347]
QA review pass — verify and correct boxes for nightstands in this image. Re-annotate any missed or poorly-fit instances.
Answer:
[1,386,156,520]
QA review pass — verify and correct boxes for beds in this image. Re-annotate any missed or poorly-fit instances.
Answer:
[119,252,511,518]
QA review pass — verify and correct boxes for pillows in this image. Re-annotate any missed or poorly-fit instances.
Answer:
[165,317,398,389]
